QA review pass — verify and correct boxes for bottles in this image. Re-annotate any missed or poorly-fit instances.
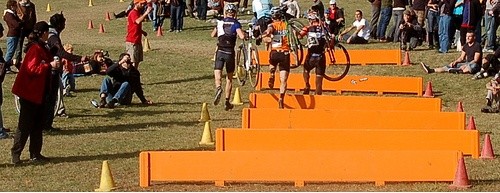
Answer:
[53,55,61,67]
[341,38,345,43]
[84,62,93,76]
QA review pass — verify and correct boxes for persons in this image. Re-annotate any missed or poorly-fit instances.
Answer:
[252,0,273,33]
[262,6,291,109]
[11,0,88,163]
[98,53,152,109]
[3,0,37,74]
[326,0,345,35]
[338,10,371,43]
[311,0,324,19]
[279,0,300,19]
[0,23,11,138]
[368,0,500,113]
[297,14,328,95]
[74,52,104,75]
[126,0,248,32]
[211,3,245,111]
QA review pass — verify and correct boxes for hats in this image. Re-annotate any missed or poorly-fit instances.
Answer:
[134,0,146,4]
[329,0,336,4]
[34,21,53,33]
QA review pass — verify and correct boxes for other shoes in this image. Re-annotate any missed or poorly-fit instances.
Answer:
[473,72,488,79]
[12,155,21,163]
[99,100,105,107]
[278,98,283,108]
[225,103,233,111]
[0,131,9,138]
[44,126,60,132]
[104,102,114,108]
[6,68,12,73]
[214,89,223,104]
[30,153,47,160]
[268,75,275,88]
[420,62,431,73]
[448,68,460,73]
[167,28,182,33]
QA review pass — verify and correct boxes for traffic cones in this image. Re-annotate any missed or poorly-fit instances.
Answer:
[105,12,110,21]
[119,0,125,2]
[88,0,94,6]
[403,52,412,66]
[94,160,121,192]
[198,103,214,122]
[448,158,473,188]
[230,87,245,105]
[47,3,51,11]
[88,21,105,33]
[237,66,243,77]
[156,26,163,38]
[479,134,497,158]
[467,116,477,130]
[423,81,434,98]
[143,38,151,52]
[457,102,464,112]
[199,122,216,145]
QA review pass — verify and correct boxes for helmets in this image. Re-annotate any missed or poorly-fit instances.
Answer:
[225,4,237,12]
[270,7,281,16]
[307,12,319,19]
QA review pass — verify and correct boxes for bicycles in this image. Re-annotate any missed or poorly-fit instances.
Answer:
[235,23,260,88]
[280,6,350,81]
